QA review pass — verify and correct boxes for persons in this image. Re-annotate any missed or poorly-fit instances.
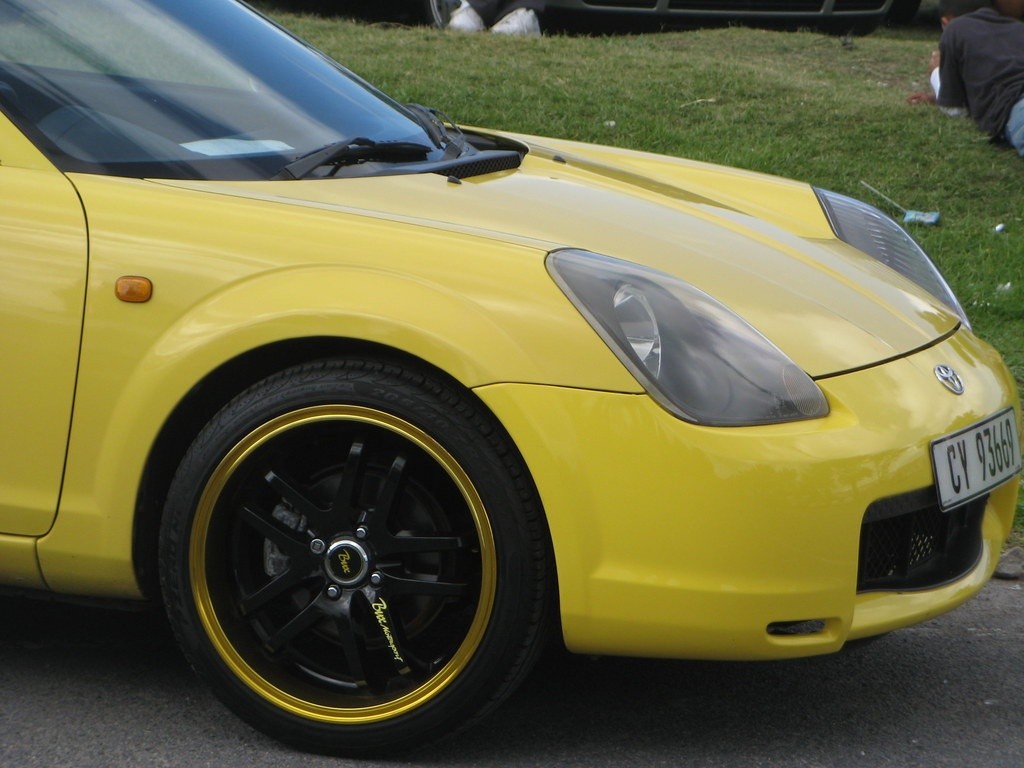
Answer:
[929,0,1024,157]
[445,0,546,37]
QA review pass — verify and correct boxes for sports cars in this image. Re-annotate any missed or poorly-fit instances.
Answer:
[1,2,1024,759]
